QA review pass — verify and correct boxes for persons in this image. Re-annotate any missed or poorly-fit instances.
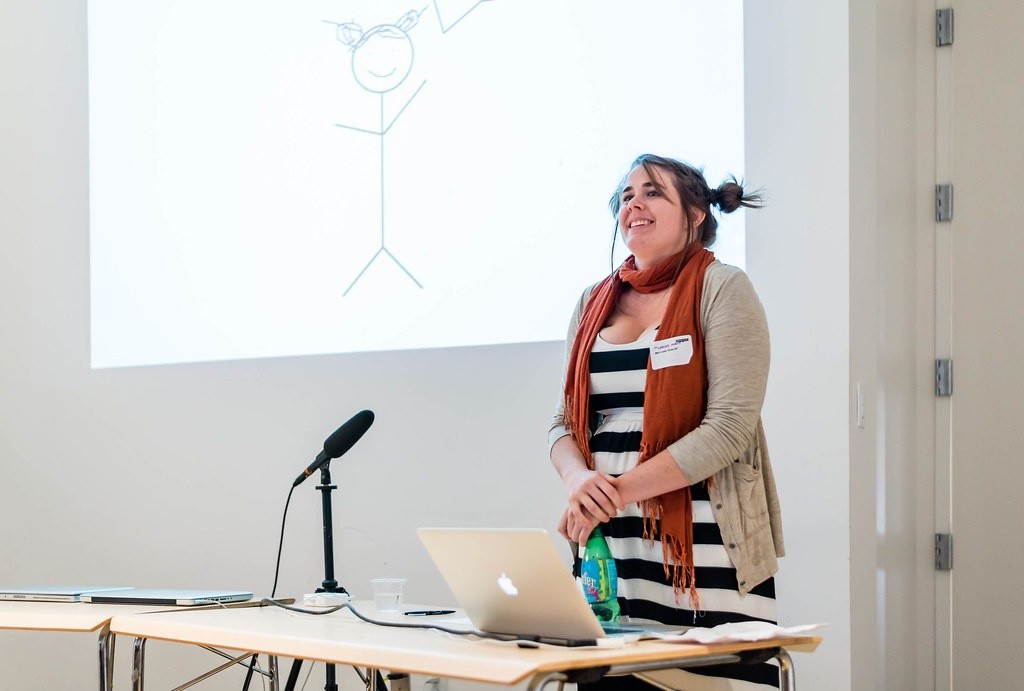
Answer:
[546,155,785,631]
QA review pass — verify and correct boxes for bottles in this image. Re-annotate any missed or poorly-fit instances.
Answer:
[580,526,620,624]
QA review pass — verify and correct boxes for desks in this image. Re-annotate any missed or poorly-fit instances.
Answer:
[0,583,819,691]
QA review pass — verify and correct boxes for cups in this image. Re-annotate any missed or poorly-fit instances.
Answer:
[371,579,404,611]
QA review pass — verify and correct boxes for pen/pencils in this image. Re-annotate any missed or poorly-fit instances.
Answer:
[401,610,457,616]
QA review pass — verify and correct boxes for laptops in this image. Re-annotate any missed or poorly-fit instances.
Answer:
[79,588,253,606]
[0,584,135,604]
[414,528,687,644]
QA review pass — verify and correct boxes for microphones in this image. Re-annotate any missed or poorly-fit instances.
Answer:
[293,410,375,488]
